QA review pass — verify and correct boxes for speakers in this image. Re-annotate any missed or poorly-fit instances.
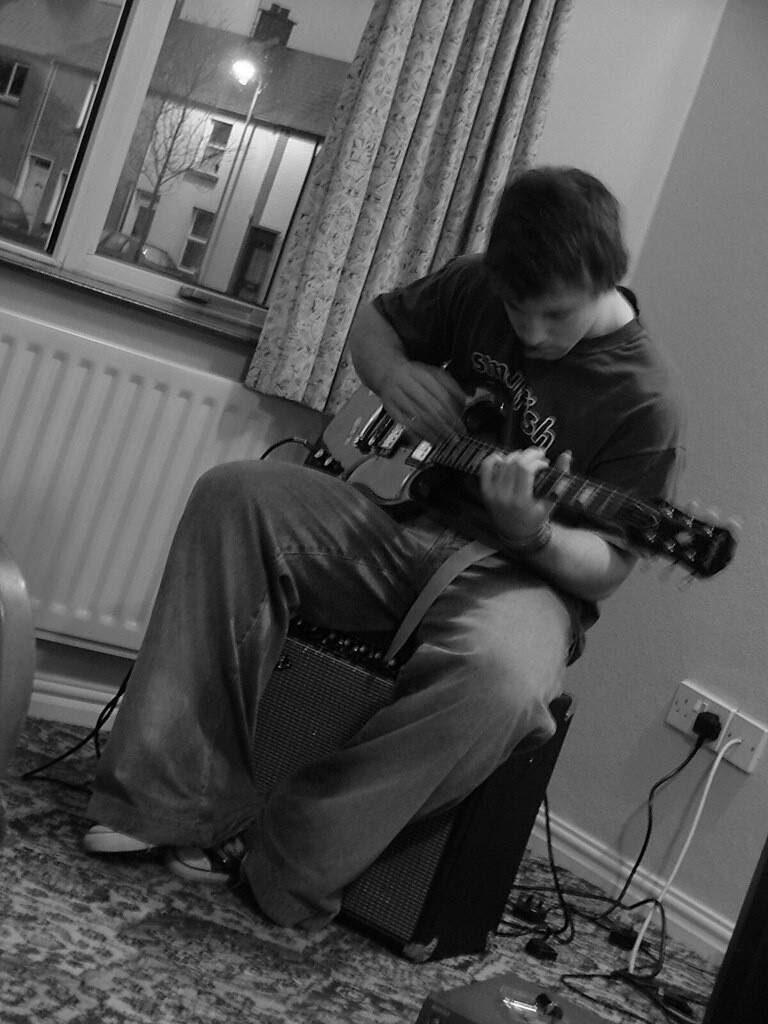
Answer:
[242,612,581,962]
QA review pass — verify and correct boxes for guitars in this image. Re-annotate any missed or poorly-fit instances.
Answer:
[319,375,744,592]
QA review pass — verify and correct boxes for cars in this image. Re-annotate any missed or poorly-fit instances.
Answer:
[41,226,184,279]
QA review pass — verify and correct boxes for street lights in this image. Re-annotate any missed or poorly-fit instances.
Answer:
[191,56,268,285]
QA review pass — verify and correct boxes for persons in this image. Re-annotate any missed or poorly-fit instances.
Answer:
[82,165,685,931]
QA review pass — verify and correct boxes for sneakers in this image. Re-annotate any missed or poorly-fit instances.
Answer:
[167,838,246,884]
[83,825,151,853]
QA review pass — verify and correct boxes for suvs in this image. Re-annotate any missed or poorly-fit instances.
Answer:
[0,194,28,244]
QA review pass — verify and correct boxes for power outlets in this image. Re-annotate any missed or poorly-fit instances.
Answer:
[664,681,736,752]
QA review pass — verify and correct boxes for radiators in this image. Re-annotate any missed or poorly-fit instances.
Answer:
[0,307,329,662]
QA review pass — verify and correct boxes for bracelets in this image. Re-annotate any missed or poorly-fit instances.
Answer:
[498,524,551,552]
